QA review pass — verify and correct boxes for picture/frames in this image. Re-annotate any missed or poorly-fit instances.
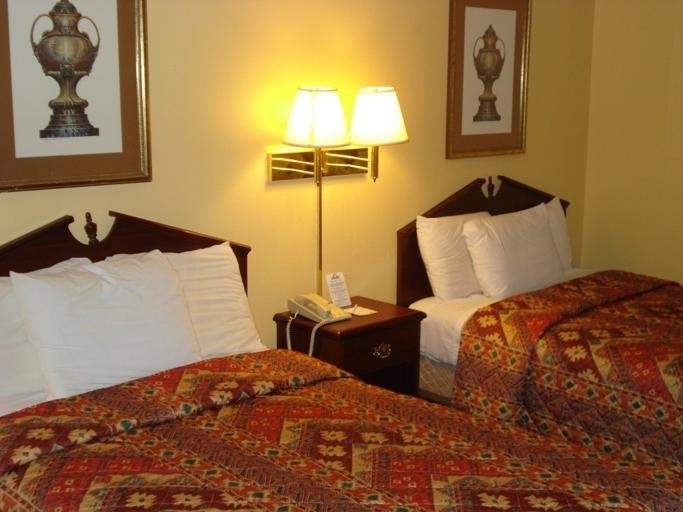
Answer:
[0,0,152,193]
[445,2,529,157]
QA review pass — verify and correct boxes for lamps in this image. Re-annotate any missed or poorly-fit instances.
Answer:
[270,85,409,183]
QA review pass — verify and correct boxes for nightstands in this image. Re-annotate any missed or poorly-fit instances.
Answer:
[272,295,428,397]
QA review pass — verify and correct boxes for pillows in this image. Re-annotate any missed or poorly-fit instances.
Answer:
[104,240,268,360]
[546,196,572,278]
[463,203,563,301]
[415,212,493,298]
[12,251,202,400]
[0,257,93,418]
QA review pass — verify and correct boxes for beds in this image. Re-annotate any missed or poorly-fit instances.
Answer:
[397,175,683,467]
[0,208,682,512]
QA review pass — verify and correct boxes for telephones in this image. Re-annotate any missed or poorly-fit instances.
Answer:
[287,293,351,323]
